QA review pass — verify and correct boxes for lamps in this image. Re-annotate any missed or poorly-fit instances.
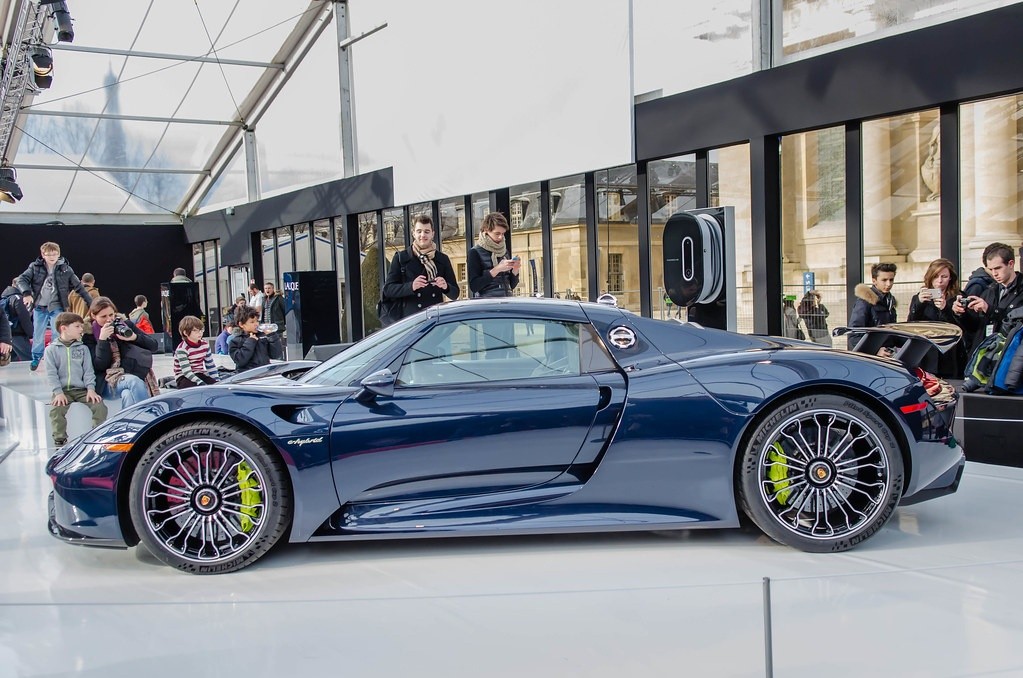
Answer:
[0,160,23,205]
[31,52,53,88]
[39,0,74,42]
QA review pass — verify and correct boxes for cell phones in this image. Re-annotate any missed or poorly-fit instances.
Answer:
[923,289,942,299]
[424,279,436,284]
[512,256,519,260]
[884,347,894,353]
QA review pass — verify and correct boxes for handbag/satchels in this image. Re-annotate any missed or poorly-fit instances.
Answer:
[375,251,406,329]
[121,345,153,380]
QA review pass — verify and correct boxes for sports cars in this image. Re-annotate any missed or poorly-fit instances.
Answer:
[44,291,967,577]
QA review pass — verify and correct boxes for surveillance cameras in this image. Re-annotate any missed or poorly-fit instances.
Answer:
[47,0,76,43]
[230,208,235,216]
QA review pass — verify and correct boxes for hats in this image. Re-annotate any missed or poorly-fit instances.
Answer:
[236,297,246,304]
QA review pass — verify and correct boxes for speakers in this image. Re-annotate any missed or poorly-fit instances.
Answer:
[283,270,341,362]
[160,282,202,357]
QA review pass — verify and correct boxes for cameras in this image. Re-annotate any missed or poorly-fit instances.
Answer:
[958,297,973,310]
[109,317,133,338]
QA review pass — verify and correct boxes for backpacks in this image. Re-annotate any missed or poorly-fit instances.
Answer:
[1,296,18,329]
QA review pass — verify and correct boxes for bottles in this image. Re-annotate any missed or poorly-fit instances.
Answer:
[255,323,278,333]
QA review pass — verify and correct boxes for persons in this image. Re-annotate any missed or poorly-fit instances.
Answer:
[467,213,521,359]
[781,242,1023,379]
[523,290,581,336]
[663,292,672,317]
[0,241,285,450]
[382,215,459,384]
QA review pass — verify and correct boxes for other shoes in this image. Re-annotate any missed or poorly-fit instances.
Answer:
[30,356,41,370]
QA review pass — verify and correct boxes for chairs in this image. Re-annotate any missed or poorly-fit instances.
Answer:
[530,322,565,377]
